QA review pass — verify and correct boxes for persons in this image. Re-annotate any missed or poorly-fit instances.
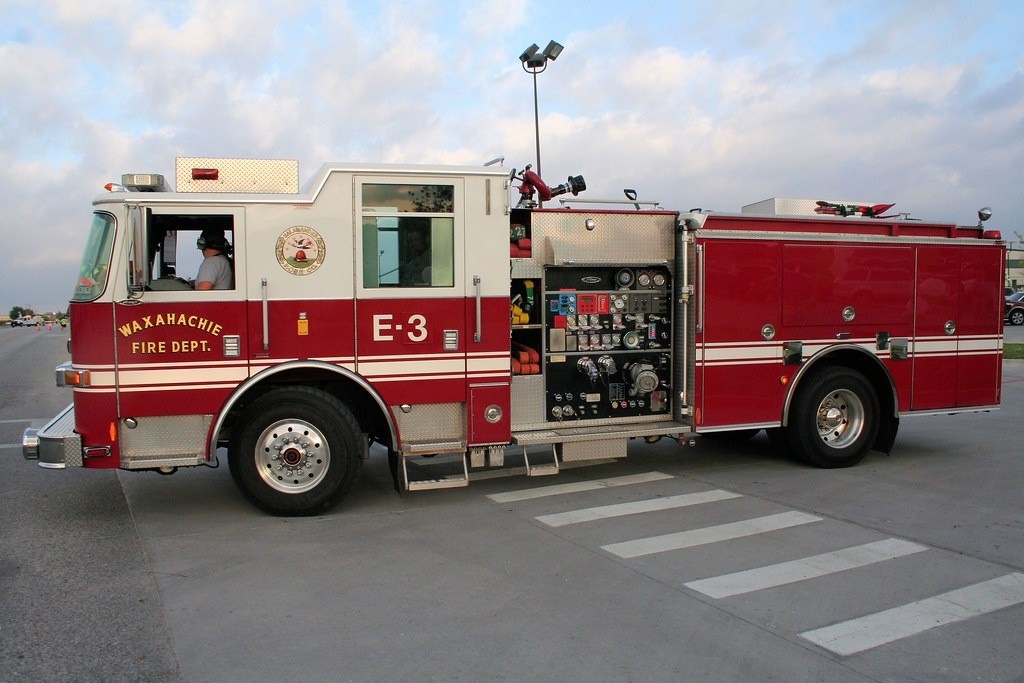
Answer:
[189,227,233,290]
[40,316,67,332]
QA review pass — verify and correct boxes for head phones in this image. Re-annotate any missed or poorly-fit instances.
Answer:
[197,237,229,250]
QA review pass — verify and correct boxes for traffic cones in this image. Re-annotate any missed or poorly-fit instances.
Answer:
[60,325,64,330]
[47,322,53,330]
[36,323,41,331]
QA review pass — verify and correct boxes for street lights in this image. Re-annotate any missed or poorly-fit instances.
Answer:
[519,40,565,208]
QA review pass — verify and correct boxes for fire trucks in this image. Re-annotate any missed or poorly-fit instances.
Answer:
[23,156,1008,518]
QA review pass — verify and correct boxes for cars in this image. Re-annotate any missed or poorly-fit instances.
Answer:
[11,316,32,327]
[1004,291,1024,326]
